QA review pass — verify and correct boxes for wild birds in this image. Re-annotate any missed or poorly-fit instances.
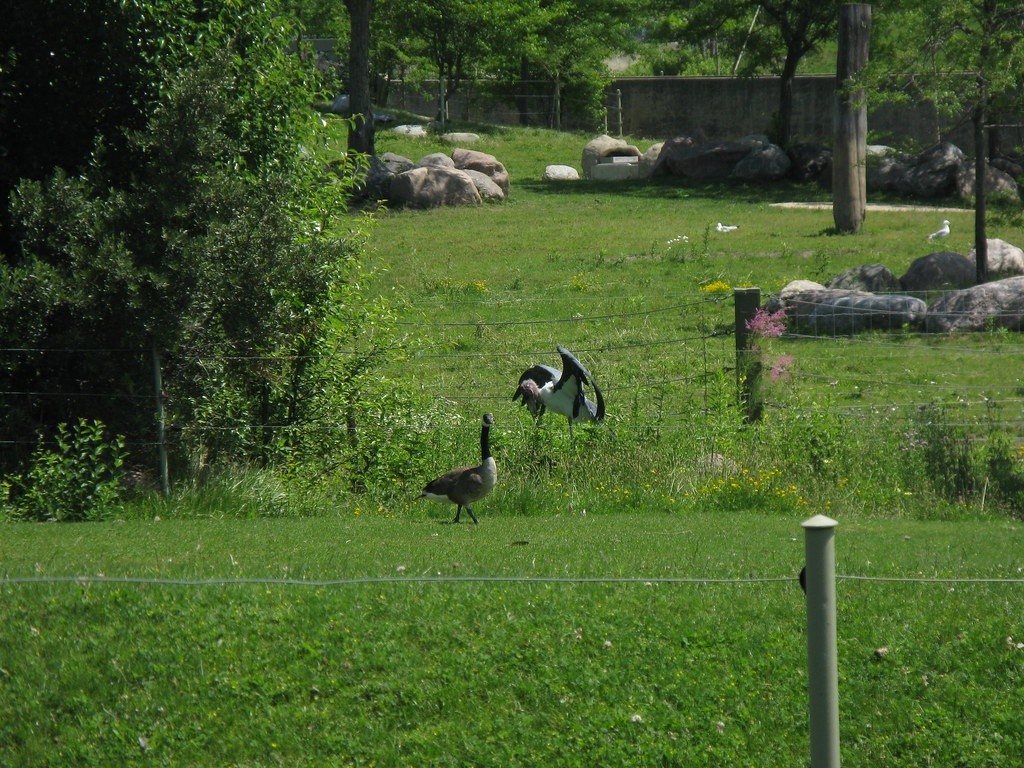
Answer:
[511,346,606,444]
[414,413,496,523]
[717,222,740,233]
[929,219,952,241]
